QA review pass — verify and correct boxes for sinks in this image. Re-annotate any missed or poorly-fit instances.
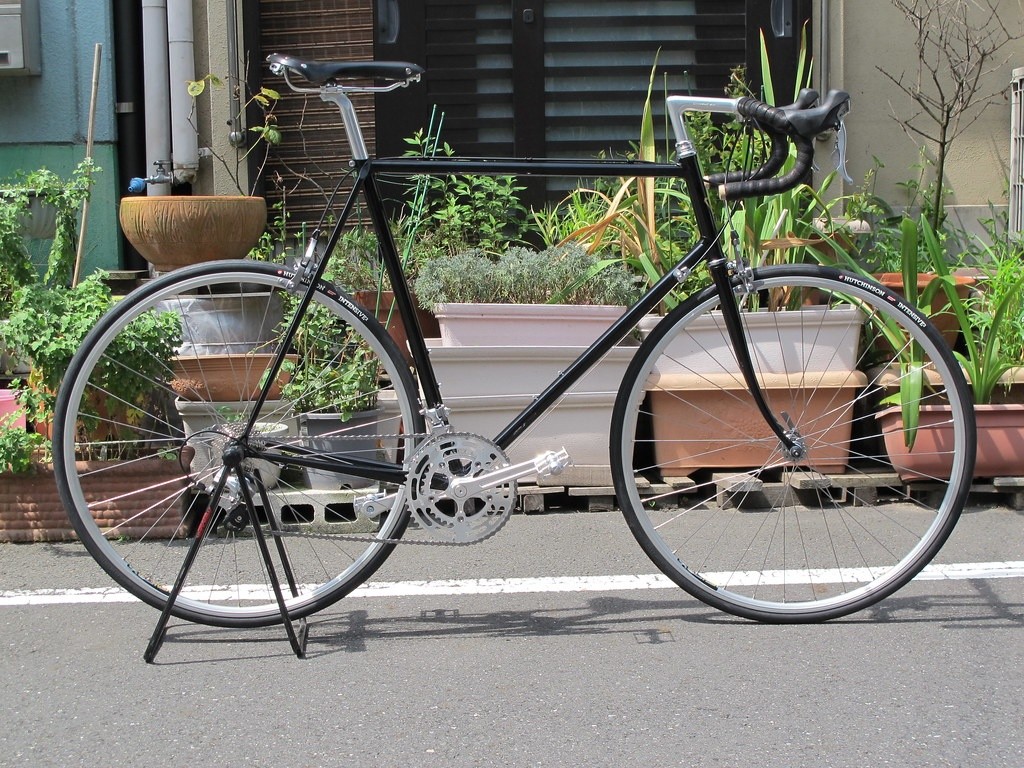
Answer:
[119,195,268,272]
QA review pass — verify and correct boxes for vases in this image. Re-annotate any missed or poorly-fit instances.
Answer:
[165,353,304,401]
[117,195,266,272]
[173,395,292,490]
[152,292,286,357]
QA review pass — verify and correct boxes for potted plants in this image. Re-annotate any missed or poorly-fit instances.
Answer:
[0,165,197,542]
[795,203,1024,483]
[266,223,386,489]
[329,15,1024,488]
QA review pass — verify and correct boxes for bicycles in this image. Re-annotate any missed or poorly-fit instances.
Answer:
[48,51,979,663]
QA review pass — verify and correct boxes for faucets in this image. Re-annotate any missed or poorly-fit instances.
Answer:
[128,159,174,193]
